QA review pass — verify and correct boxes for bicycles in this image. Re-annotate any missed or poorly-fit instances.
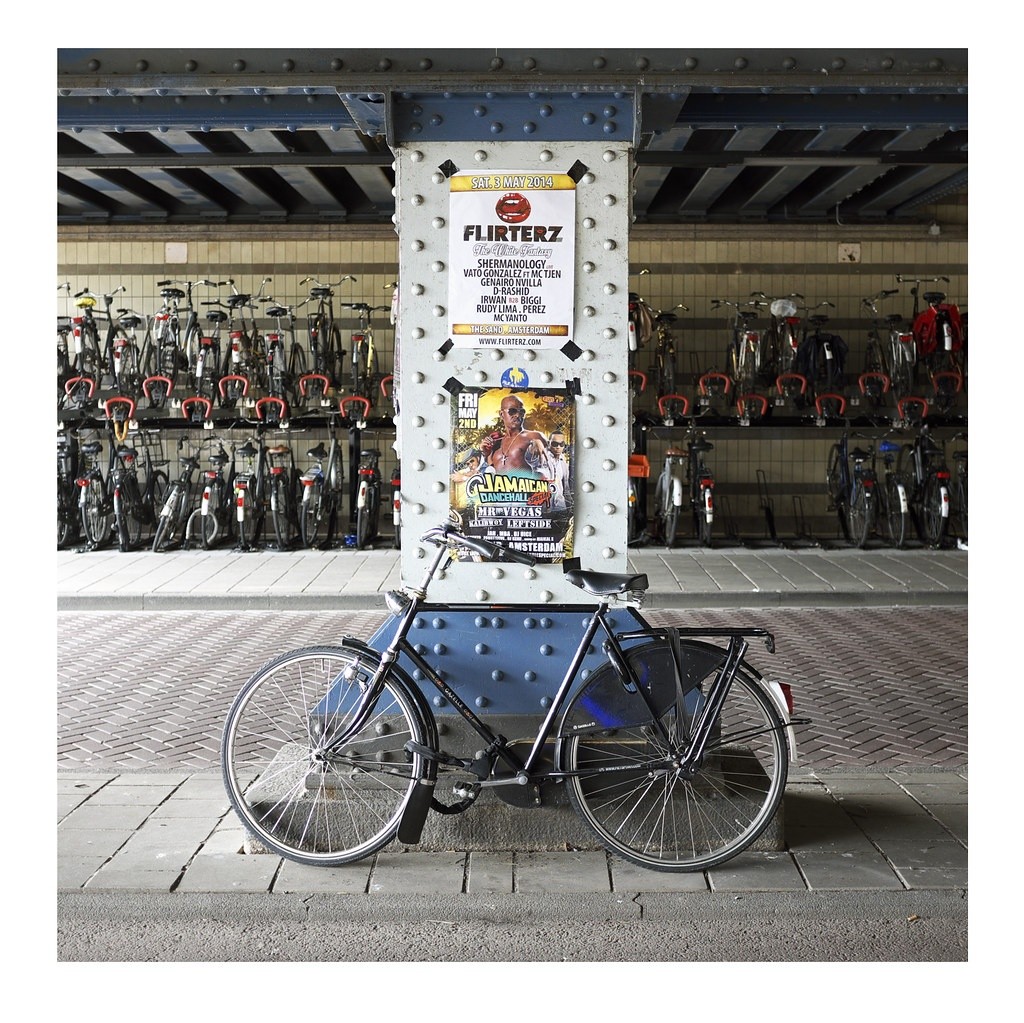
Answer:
[59,274,405,552]
[629,268,970,550]
[216,520,816,872]
